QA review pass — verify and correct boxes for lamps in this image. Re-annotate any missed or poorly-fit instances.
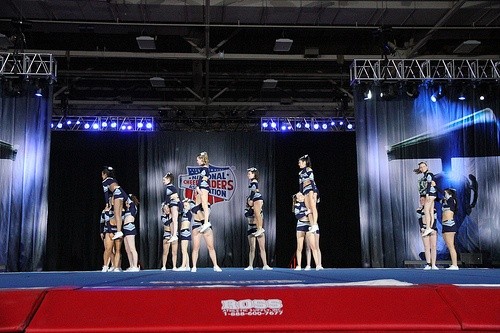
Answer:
[259,116,357,133]
[273,38,293,52]
[51,115,162,132]
[151,76,166,88]
[136,36,157,49]
[0,75,53,97]
[263,79,278,88]
[358,79,498,102]
[385,41,397,52]
[452,40,481,55]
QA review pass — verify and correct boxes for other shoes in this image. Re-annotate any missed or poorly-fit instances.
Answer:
[244,266,253,270]
[306,225,319,233]
[108,266,115,271]
[124,267,140,272]
[214,265,222,272]
[294,266,301,270]
[423,264,431,270]
[161,266,166,271]
[422,228,433,236]
[198,223,211,232]
[102,266,109,272]
[252,228,265,237]
[191,267,196,272]
[112,231,123,239]
[445,265,459,270]
[167,235,178,242]
[263,265,273,270]
[316,264,323,271]
[177,267,190,271]
[432,266,439,270]
[304,266,310,271]
[172,267,177,271]
[113,268,120,272]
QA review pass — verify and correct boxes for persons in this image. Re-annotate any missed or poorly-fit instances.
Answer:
[189,191,223,272]
[161,201,179,271]
[178,198,192,271]
[162,172,181,242]
[195,151,211,233]
[435,188,459,270]
[99,195,124,272]
[123,193,141,272]
[247,167,265,237]
[412,161,438,237]
[101,166,128,240]
[292,192,324,271]
[244,196,273,271]
[416,195,439,270]
[298,154,320,233]
